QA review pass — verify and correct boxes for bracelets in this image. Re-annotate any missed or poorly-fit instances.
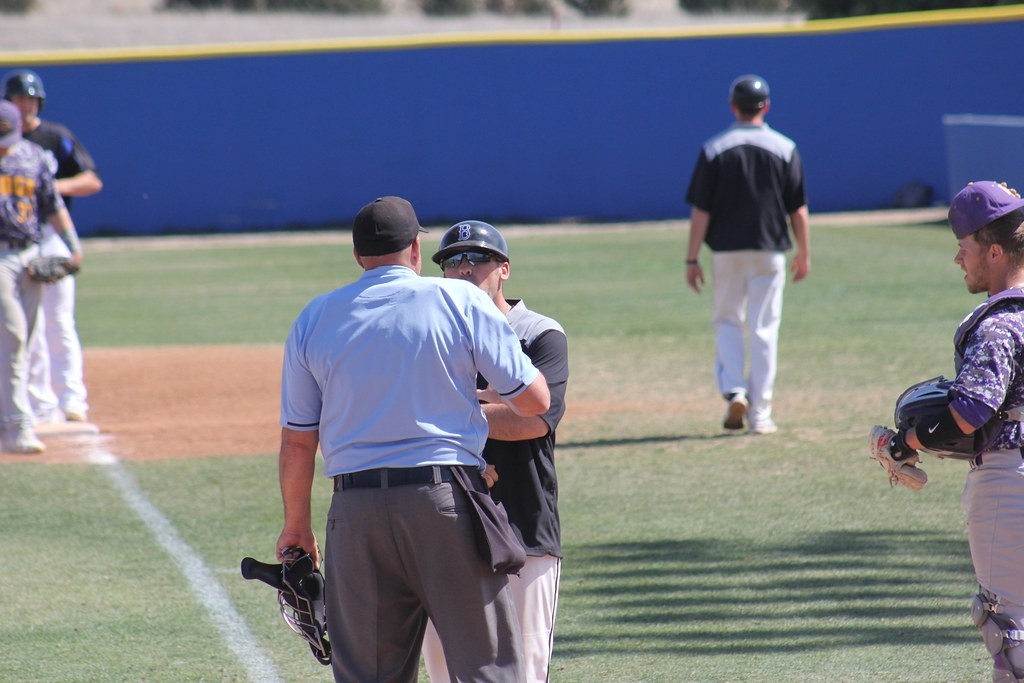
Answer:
[915,408,967,451]
[686,259,698,265]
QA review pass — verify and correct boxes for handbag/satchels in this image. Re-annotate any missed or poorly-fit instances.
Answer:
[450,466,527,580]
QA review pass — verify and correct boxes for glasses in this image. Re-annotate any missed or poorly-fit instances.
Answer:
[440,248,503,268]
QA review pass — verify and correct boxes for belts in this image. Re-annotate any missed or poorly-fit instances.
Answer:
[332,465,483,488]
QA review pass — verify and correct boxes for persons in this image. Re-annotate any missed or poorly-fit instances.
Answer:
[422,220,570,683]
[888,183,1024,683]
[275,195,551,683]
[0,69,103,455]
[684,76,811,436]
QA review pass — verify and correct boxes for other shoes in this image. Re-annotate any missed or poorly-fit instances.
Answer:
[65,405,87,422]
[35,409,65,423]
[722,391,749,430]
[749,417,776,434]
[0,422,45,453]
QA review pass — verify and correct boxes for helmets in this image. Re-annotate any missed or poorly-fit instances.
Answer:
[893,375,1005,461]
[731,78,769,111]
[431,221,509,264]
[3,72,46,104]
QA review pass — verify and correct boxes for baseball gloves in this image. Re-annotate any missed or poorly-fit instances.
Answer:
[27,254,80,280]
[867,423,928,491]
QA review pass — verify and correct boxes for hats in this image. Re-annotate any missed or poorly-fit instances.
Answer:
[948,180,1024,240]
[352,196,428,256]
[0,99,22,148]
